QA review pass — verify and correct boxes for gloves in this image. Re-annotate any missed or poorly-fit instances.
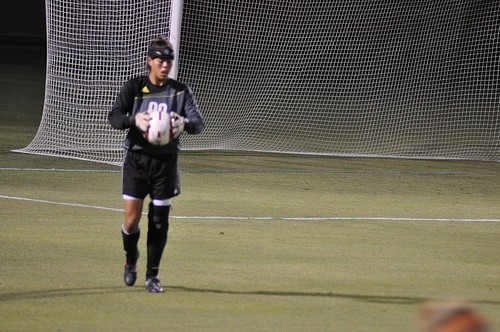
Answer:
[170,111,187,140]
[135,111,152,132]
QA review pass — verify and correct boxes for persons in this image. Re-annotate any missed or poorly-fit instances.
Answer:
[109,40,206,293]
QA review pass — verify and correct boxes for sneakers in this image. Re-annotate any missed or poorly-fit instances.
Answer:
[145,277,164,293]
[124,258,137,286]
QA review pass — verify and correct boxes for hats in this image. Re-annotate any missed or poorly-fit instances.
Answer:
[147,47,174,59]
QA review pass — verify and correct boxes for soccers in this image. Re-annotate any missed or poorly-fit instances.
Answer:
[143,112,176,145]
